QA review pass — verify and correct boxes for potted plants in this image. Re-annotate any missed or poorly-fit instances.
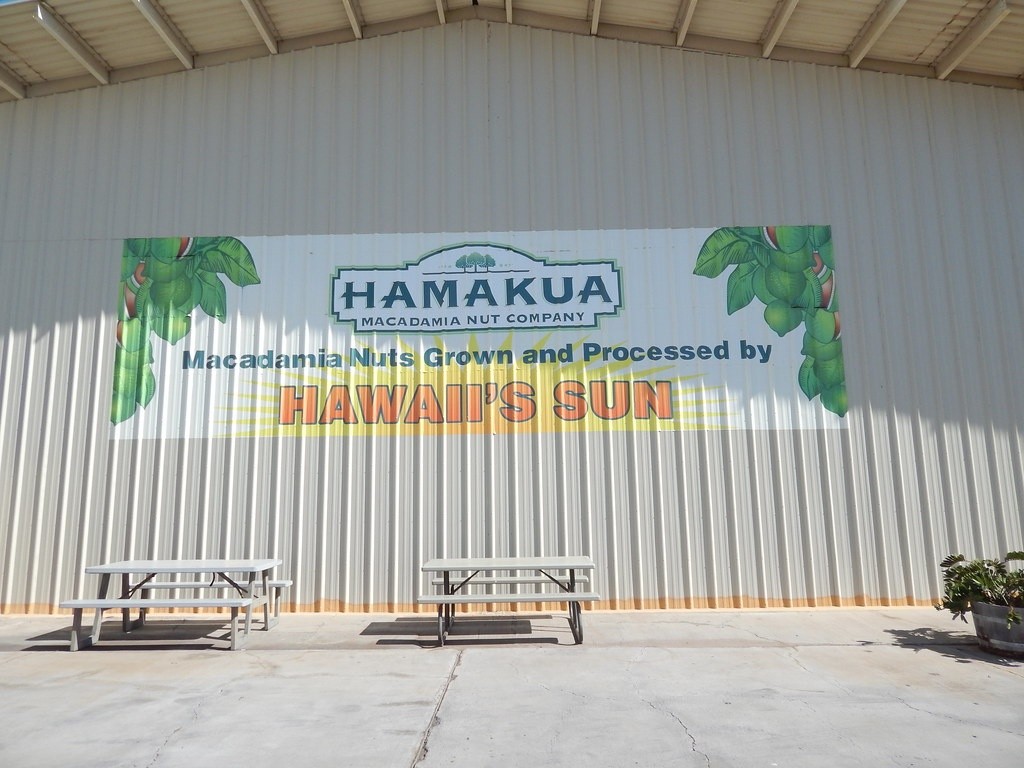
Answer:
[935,550,1024,657]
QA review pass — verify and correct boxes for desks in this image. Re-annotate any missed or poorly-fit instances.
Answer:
[85,560,284,646]
[421,556,594,646]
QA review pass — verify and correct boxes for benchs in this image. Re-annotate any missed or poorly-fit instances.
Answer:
[418,592,602,646]
[432,575,588,625]
[129,580,293,630]
[59,598,253,651]
